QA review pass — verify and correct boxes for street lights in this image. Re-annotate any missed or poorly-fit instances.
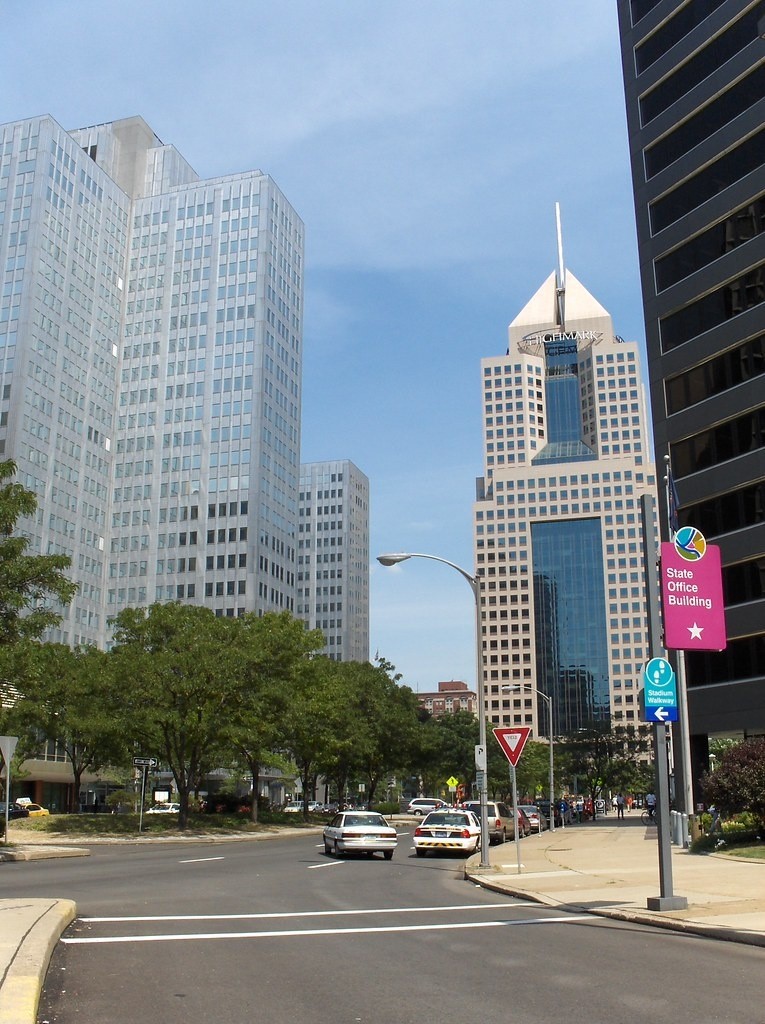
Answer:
[500,687,554,832]
[375,553,490,869]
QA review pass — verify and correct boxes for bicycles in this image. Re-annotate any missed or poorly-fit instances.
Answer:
[640,807,658,826]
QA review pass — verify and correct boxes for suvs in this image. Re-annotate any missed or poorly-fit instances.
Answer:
[564,794,584,815]
[407,797,450,816]
[532,797,562,827]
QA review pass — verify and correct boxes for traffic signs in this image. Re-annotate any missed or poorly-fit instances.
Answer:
[643,658,677,722]
[132,757,157,768]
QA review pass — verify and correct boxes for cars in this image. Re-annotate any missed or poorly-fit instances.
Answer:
[508,807,531,838]
[413,807,490,858]
[517,805,548,833]
[146,802,181,814]
[284,801,305,813]
[0,802,29,821]
[15,797,49,817]
[312,804,340,814]
[308,800,322,812]
[323,810,398,860]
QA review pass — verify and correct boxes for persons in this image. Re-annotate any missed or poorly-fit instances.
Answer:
[708,803,723,835]
[617,793,625,819]
[645,791,656,820]
[625,795,632,812]
[611,794,618,812]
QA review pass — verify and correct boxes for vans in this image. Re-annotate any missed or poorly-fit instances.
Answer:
[463,800,515,844]
